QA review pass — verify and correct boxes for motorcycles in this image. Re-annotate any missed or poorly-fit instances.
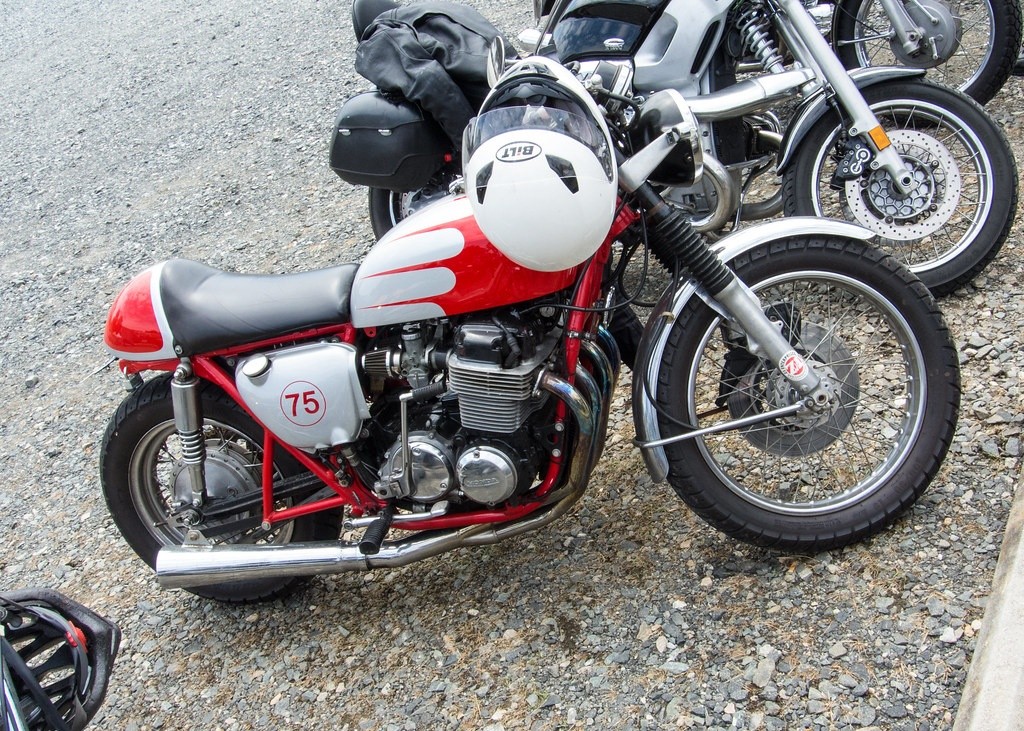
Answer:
[331,0,1024,301]
[100,38,963,605]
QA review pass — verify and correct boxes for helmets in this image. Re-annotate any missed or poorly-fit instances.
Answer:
[461,55,619,272]
